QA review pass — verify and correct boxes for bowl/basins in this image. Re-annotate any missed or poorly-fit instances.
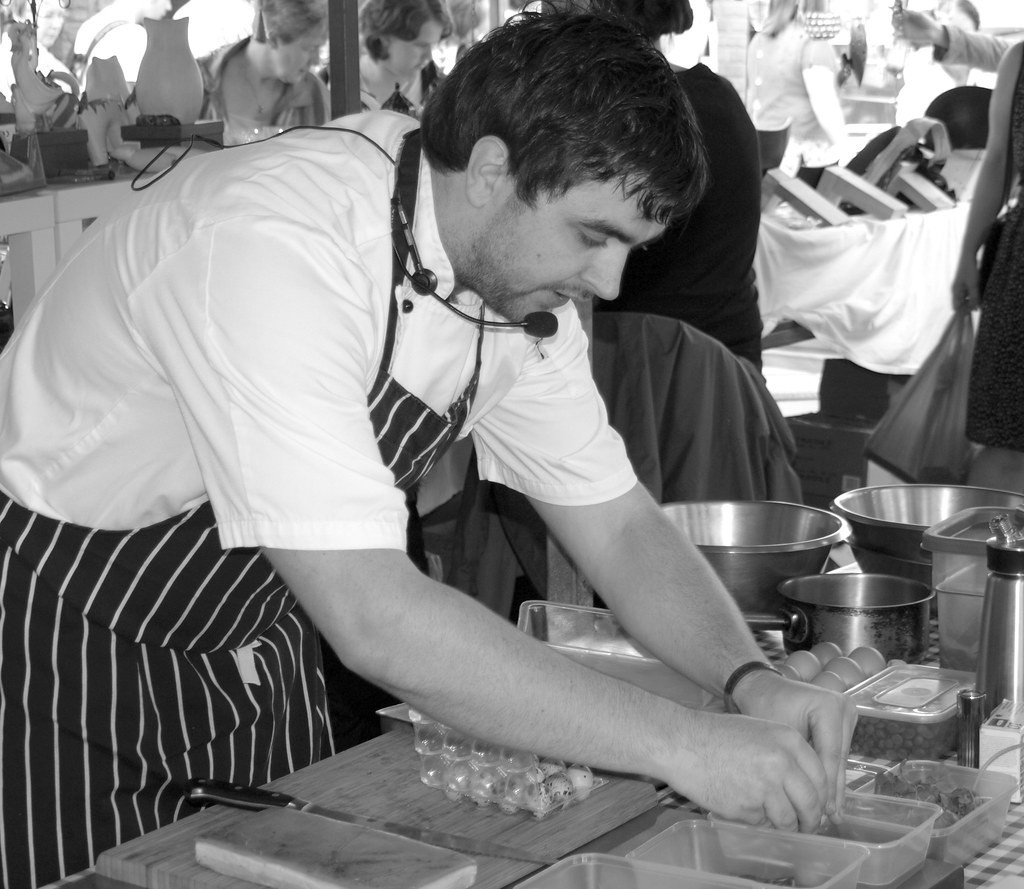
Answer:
[829,484,1024,564]
[655,500,853,612]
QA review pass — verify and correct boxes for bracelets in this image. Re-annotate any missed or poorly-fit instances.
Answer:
[722,660,784,715]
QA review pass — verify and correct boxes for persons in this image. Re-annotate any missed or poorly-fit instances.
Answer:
[0,0,1023,504]
[0,8,864,889]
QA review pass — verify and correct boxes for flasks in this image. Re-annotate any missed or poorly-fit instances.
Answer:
[975,536,1024,715]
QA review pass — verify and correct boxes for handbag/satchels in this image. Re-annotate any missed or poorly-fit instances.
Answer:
[863,302,974,484]
[972,208,1010,299]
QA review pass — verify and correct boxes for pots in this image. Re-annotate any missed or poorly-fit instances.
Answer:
[744,573,936,663]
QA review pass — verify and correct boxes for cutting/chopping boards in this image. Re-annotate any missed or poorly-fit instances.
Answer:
[95,721,658,888]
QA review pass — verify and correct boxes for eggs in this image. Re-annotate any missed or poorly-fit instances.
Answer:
[771,642,886,695]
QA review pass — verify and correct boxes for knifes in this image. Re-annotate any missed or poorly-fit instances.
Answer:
[185,777,559,865]
